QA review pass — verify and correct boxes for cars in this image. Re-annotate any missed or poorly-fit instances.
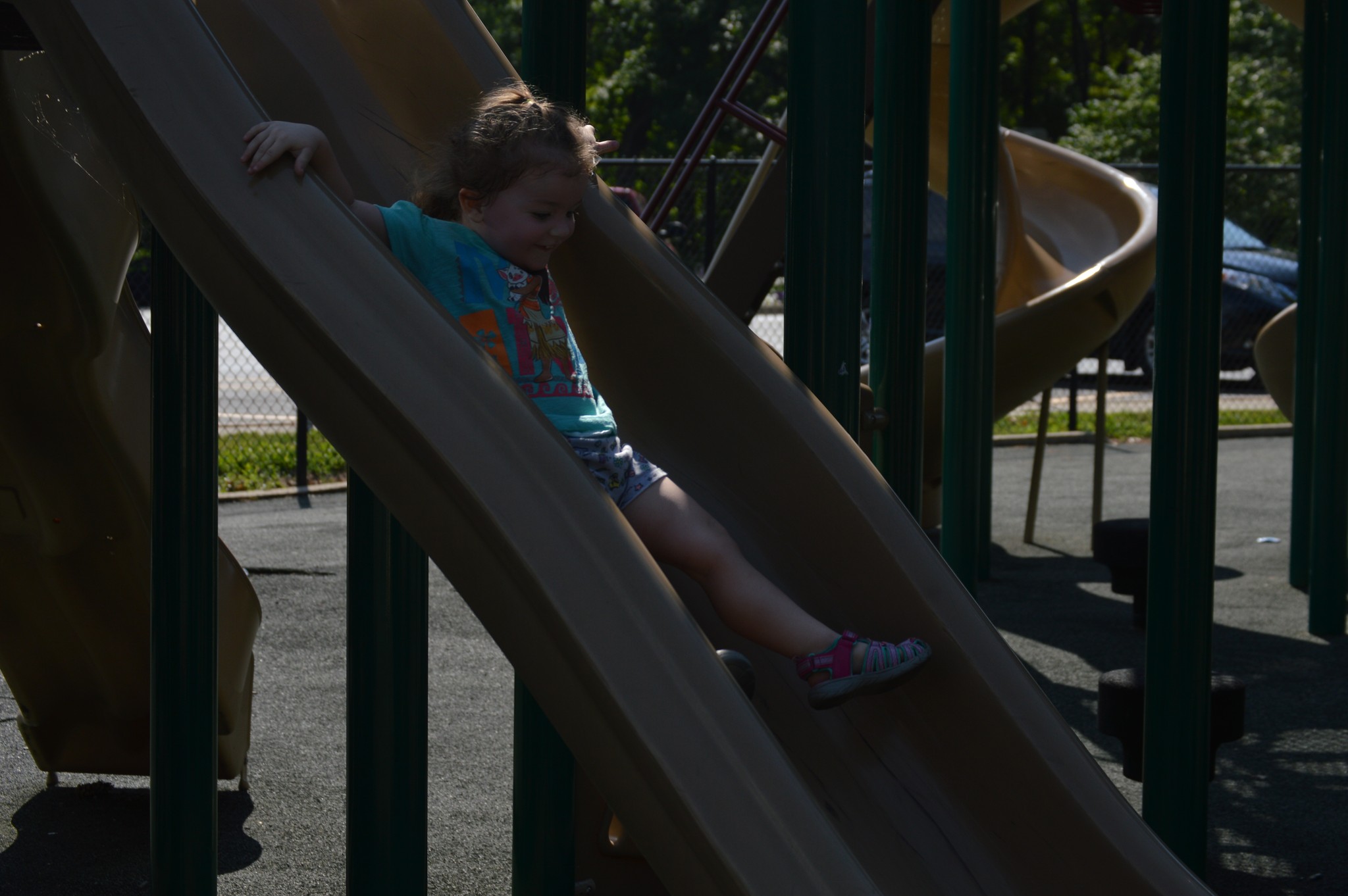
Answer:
[1060,183,1300,384]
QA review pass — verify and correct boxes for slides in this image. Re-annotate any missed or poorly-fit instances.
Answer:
[0,0,1296,896]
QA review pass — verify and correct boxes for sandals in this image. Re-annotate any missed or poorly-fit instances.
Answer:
[798,632,930,699]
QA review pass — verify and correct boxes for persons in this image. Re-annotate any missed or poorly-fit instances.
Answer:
[240,81,931,705]
